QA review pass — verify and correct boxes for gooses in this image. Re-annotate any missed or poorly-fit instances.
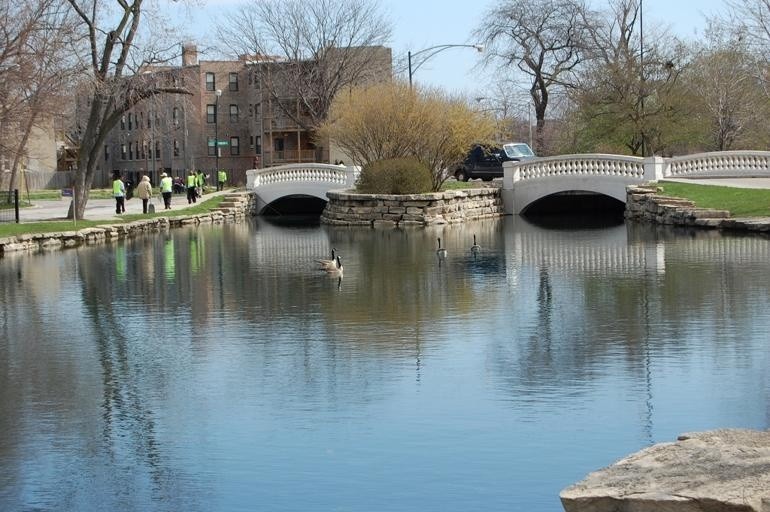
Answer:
[471,233,481,253]
[436,238,447,255]
[323,272,343,293]
[312,248,344,273]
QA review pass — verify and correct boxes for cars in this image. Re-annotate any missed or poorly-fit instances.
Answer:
[448,141,539,182]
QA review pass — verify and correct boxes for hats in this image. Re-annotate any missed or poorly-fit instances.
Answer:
[160,172,168,177]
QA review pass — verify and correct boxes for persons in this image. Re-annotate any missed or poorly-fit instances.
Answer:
[174,178,185,194]
[186,168,207,205]
[215,167,227,192]
[159,172,174,210]
[112,174,127,217]
[252,156,260,170]
[137,175,153,215]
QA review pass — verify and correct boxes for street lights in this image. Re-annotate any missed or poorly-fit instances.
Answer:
[408,43,484,90]
[214,89,222,191]
